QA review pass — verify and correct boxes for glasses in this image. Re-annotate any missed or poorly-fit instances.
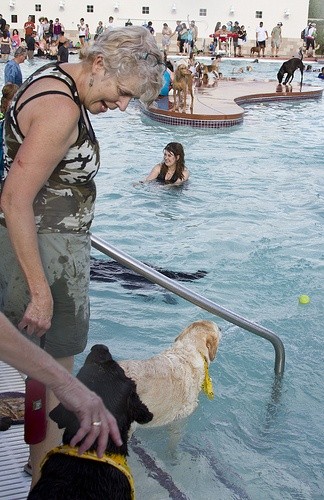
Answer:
[116,47,167,75]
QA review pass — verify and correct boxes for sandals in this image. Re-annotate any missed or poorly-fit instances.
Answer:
[24,457,33,476]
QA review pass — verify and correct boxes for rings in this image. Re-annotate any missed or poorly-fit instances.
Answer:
[92,422,101,426]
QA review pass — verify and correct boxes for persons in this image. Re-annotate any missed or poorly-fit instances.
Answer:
[147,142,189,184]
[256,22,283,57]
[209,21,247,57]
[301,64,324,80]
[125,19,154,35]
[105,16,113,31]
[298,22,318,58]
[0,26,167,491]
[0,84,19,117]
[168,15,199,55]
[162,23,172,55]
[96,21,104,37]
[0,309,122,459]
[0,14,73,63]
[5,47,27,87]
[74,18,89,48]
[158,51,258,96]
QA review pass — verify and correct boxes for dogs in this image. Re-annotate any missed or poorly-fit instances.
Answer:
[198,64,219,80]
[26,343,154,500]
[115,318,221,441]
[277,57,305,86]
[249,45,264,57]
[168,64,194,112]
